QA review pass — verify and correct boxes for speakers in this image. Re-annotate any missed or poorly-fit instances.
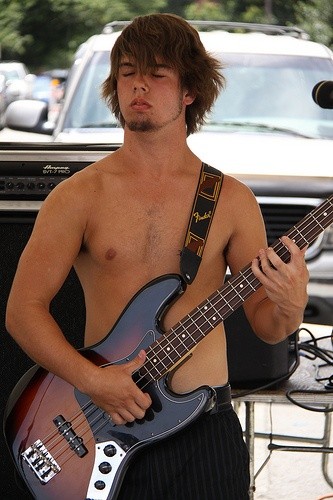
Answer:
[0,141,123,499]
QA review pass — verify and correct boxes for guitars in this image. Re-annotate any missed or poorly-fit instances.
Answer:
[4,195,332,500]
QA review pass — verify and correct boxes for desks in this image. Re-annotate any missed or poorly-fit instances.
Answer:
[229,322,333,500]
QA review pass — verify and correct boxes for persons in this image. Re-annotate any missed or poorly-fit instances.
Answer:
[4,12,311,500]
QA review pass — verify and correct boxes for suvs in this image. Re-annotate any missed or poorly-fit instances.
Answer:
[0,15,332,326]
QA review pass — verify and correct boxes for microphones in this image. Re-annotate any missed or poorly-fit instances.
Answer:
[311,80,333,109]
